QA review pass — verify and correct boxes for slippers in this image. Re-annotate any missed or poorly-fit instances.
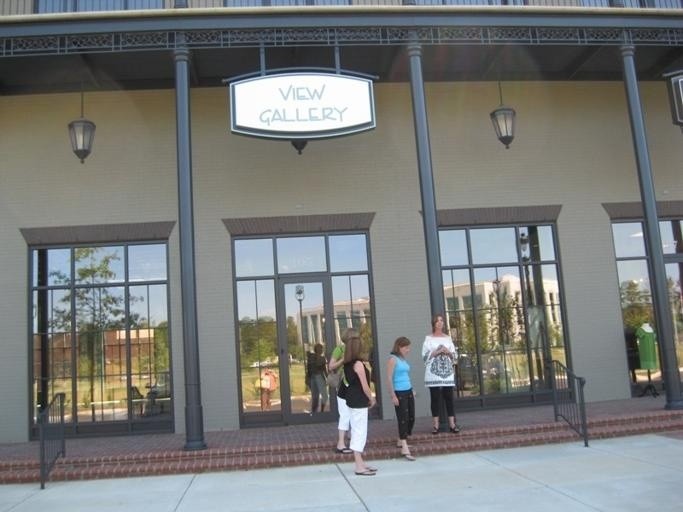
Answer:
[400,451,415,461]
[335,447,353,454]
[355,466,377,475]
[396,443,414,448]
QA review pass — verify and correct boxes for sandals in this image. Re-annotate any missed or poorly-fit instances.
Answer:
[450,424,459,432]
[431,426,440,434]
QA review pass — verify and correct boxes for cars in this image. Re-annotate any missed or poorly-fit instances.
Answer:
[249,353,308,369]
[144,370,169,407]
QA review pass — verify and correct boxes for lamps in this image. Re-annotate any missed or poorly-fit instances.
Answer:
[489,59,515,149]
[67,61,96,163]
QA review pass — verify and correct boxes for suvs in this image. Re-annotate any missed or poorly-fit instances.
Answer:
[456,353,507,392]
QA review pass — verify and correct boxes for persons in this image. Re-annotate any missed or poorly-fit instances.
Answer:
[328,328,360,454]
[345,338,377,476]
[636,322,658,371]
[306,344,328,415]
[262,366,275,410]
[386,336,416,461]
[422,314,461,434]
[625,323,641,369]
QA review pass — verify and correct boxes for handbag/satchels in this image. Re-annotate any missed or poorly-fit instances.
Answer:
[326,371,341,387]
[255,380,266,389]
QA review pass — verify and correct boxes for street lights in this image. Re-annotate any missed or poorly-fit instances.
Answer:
[296,284,309,371]
